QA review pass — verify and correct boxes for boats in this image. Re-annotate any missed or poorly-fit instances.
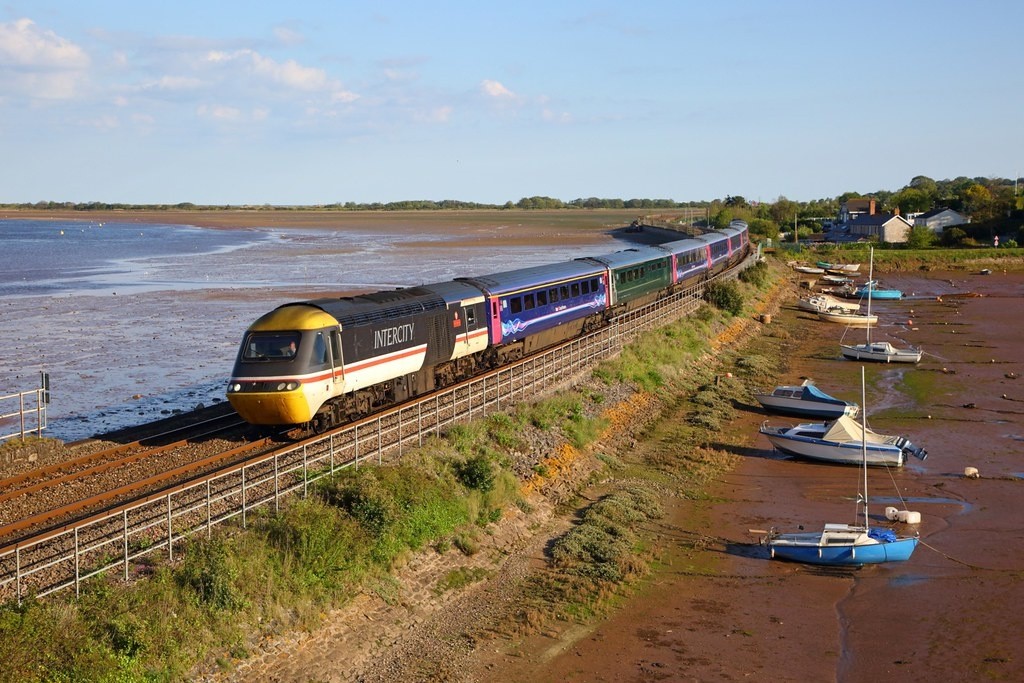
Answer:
[800,257,862,316]
[755,379,865,424]
[757,413,937,476]
[832,277,903,302]
[816,304,880,328]
[796,267,825,277]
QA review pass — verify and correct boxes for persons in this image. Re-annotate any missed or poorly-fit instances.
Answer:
[286,340,296,356]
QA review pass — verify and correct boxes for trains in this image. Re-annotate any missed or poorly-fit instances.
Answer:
[227,216,751,442]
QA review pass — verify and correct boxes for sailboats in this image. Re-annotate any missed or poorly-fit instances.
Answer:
[744,363,936,570]
[837,248,934,369]
